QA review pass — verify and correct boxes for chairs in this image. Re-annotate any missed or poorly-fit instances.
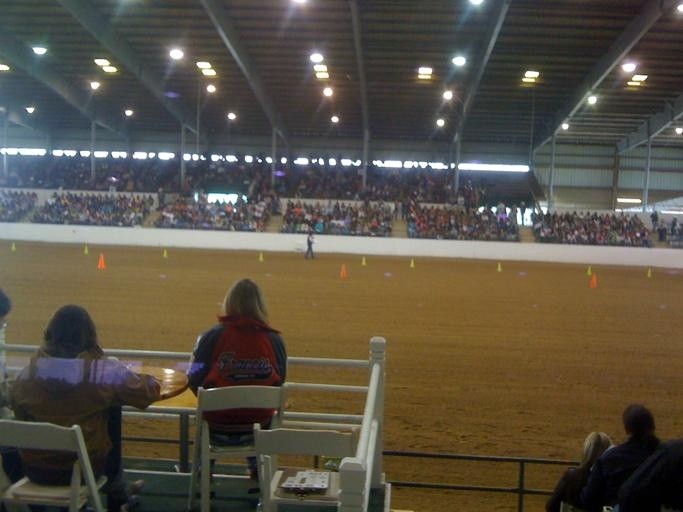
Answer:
[186,383,358,511]
[0,419,108,512]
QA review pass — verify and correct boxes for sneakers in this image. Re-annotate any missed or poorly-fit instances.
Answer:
[103,478,145,512]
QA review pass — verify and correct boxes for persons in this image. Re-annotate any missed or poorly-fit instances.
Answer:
[616,436,682,512]
[530,207,681,247]
[185,276,286,498]
[2,151,527,241]
[9,303,160,511]
[303,230,314,259]
[578,404,659,512]
[0,287,27,483]
[545,430,611,512]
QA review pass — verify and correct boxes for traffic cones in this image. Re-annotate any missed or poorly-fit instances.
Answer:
[406,257,417,269]
[585,265,598,288]
[10,240,17,251]
[94,251,106,269]
[359,254,368,268]
[337,262,350,279]
[257,250,265,262]
[82,242,88,254]
[161,248,168,257]
[496,262,501,272]
[646,267,652,277]
